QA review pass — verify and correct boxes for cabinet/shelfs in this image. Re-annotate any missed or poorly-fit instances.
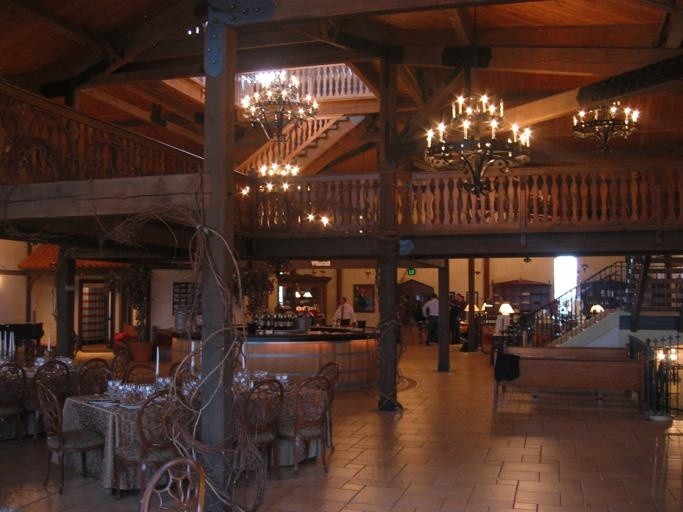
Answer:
[253,309,298,333]
[284,302,320,314]
[509,317,512,325]
[482,314,496,326]
[491,277,552,315]
[534,314,552,330]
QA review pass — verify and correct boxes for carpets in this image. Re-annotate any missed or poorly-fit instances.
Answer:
[341,307,343,319]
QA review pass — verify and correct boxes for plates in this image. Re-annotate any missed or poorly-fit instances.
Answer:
[491,277,552,315]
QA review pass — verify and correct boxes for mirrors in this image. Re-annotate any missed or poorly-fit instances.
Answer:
[279,285,320,313]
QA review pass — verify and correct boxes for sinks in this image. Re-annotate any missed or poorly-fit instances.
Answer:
[109,324,173,360]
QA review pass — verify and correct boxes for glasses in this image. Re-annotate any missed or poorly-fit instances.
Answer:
[497,301,516,316]
[423,68,532,196]
[242,68,317,141]
[292,290,301,308]
[300,288,312,308]
[467,305,478,316]
[589,303,607,316]
[569,98,639,156]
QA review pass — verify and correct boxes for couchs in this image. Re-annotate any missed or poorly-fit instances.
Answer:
[109,324,173,360]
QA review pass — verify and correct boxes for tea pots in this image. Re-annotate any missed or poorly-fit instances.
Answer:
[279,285,320,313]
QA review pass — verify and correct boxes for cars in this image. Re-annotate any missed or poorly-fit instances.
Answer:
[102,370,291,404]
[0,355,76,376]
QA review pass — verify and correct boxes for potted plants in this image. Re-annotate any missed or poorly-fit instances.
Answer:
[353,284,375,313]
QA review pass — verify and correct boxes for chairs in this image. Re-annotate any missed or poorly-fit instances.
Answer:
[0,355,337,494]
[141,456,206,512]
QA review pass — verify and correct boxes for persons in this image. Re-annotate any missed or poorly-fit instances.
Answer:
[396,292,467,346]
[332,297,358,328]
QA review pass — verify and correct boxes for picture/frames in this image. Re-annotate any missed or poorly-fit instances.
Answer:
[353,284,375,313]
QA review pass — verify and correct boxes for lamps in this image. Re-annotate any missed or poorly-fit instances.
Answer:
[242,68,317,141]
[569,98,639,156]
[423,68,532,196]
[141,456,206,512]
[497,301,516,316]
[292,290,301,308]
[300,288,312,308]
[589,303,607,316]
[467,305,478,316]
